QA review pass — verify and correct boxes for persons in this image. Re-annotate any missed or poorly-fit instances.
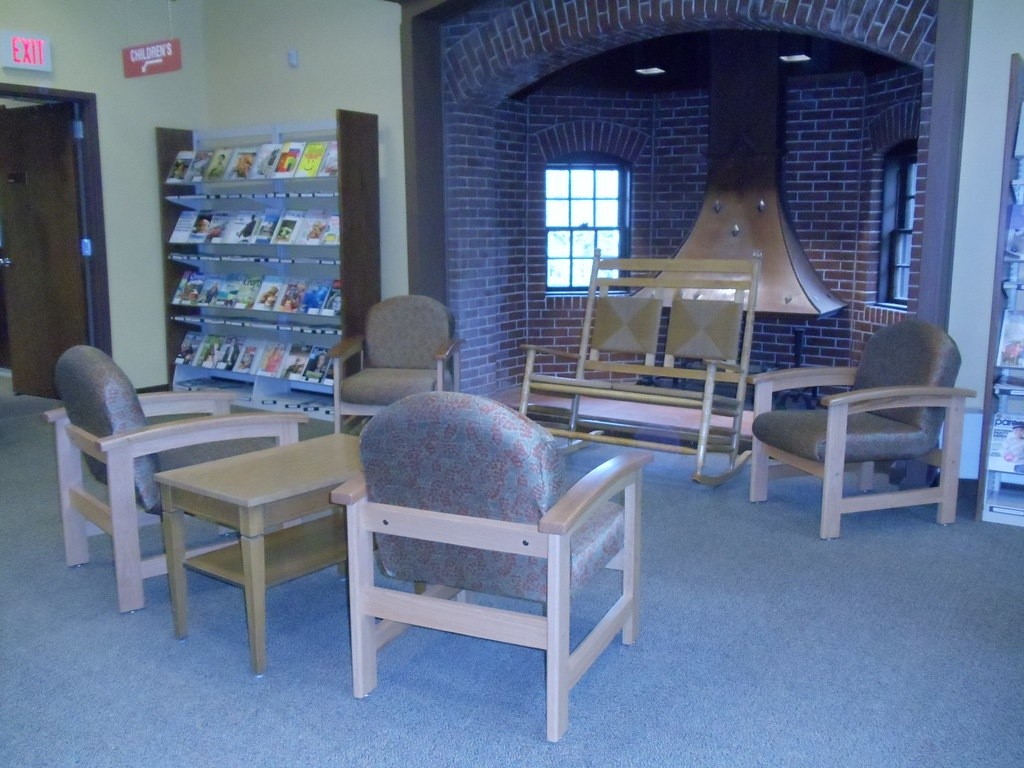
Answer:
[239,214,256,238]
[208,154,225,179]
[194,219,210,232]
[331,294,341,318]
[305,353,326,383]
[1004,421,1024,462]
[222,338,239,372]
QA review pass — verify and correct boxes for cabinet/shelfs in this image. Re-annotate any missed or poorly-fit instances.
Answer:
[975,53,1024,529]
[155,108,382,429]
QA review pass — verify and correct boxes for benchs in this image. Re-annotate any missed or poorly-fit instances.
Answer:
[522,249,761,486]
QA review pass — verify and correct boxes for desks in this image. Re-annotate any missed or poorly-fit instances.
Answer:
[154,434,427,676]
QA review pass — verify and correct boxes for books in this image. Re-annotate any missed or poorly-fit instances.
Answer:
[175,331,334,386]
[987,179,1024,475]
[171,270,341,318]
[169,210,340,247]
[167,142,338,185]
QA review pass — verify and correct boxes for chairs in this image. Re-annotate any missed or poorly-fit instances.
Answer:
[42,344,309,614]
[743,320,977,540]
[328,294,467,436]
[330,391,654,743]
